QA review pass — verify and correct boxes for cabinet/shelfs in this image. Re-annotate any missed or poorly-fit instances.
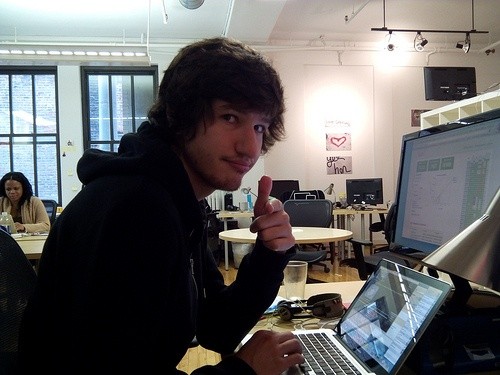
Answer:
[420,89,500,129]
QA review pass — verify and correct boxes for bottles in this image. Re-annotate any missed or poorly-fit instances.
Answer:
[0,212,10,233]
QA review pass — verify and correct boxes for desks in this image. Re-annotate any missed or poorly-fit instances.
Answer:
[4,232,49,259]
[219,207,500,375]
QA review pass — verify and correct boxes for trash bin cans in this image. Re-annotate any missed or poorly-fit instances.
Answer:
[232,242,253,270]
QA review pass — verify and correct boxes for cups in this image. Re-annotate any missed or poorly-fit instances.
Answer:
[284,261,307,300]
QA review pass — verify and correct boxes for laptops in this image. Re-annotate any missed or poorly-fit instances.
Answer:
[238,258,453,375]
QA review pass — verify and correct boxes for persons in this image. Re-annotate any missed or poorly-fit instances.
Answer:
[0,172,51,233]
[18,36,305,375]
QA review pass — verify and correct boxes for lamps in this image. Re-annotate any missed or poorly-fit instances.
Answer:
[382,30,398,52]
[413,32,429,51]
[456,33,471,54]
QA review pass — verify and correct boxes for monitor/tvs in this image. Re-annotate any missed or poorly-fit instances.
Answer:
[424,66,476,101]
[259,180,299,203]
[346,178,383,204]
[388,108,500,260]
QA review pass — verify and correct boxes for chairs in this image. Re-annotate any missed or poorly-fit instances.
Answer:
[39,199,57,226]
[340,201,424,281]
[279,200,334,273]
[0,228,38,375]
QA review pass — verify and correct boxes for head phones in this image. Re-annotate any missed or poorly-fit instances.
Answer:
[276,293,343,321]
